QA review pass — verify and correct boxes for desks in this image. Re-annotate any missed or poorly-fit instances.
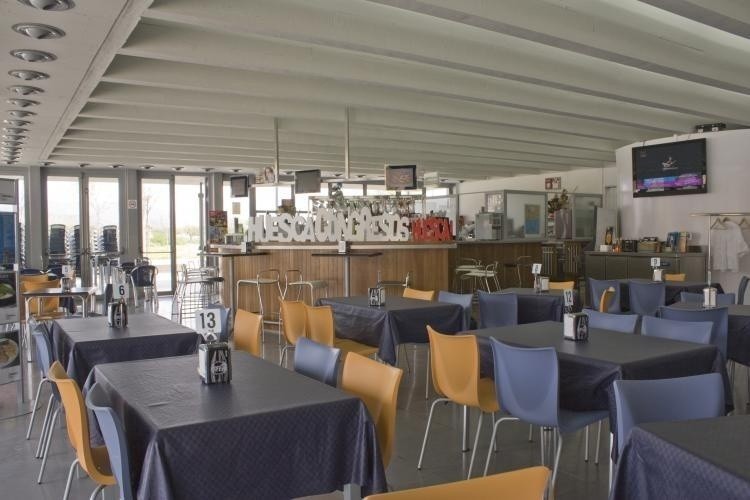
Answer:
[200,251,271,325]
[620,412,750,500]
[665,298,750,368]
[314,251,384,299]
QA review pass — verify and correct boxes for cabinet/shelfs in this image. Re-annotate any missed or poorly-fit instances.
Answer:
[588,251,711,285]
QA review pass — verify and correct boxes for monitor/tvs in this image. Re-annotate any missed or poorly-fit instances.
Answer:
[293,169,321,195]
[630,136,709,199]
[228,175,251,197]
[383,163,420,191]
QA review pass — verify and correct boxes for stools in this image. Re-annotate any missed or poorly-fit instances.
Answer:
[457,254,543,295]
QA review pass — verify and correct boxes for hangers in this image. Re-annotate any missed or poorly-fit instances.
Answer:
[708,212,750,233]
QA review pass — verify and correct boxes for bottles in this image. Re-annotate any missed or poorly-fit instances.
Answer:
[612,237,623,253]
